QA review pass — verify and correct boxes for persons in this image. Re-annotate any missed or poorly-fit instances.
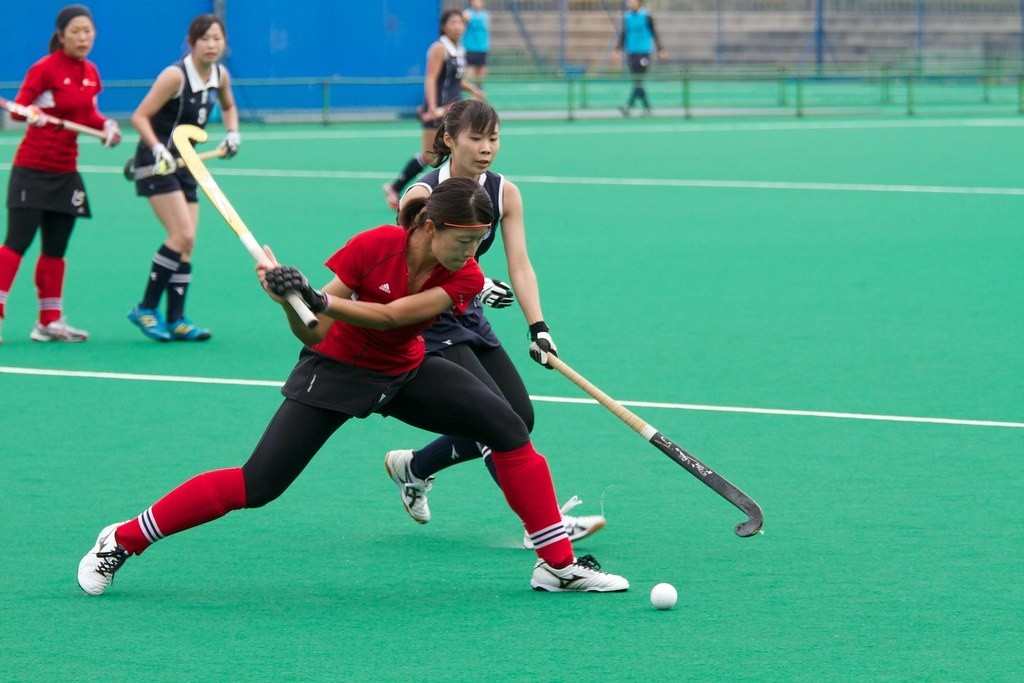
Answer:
[457,0,492,93]
[384,10,484,211]
[127,15,240,342]
[614,0,665,117]
[77,178,629,596]
[385,99,605,550]
[0,8,121,342]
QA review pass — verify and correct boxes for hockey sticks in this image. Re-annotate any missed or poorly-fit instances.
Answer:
[172,122,321,331]
[0,98,108,140]
[529,341,765,539]
[122,148,224,182]
[415,97,477,124]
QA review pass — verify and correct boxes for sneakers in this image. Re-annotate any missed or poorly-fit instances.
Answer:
[383,183,399,210]
[164,317,211,341]
[78,520,131,595]
[523,495,604,549]
[385,449,435,524]
[127,303,172,341]
[530,554,629,591]
[31,316,88,342]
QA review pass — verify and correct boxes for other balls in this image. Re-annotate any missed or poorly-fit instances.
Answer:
[649,581,679,611]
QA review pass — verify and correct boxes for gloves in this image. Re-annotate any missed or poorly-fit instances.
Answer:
[152,143,176,175]
[265,267,328,313]
[529,321,557,369]
[220,131,241,159]
[473,278,513,308]
[101,119,121,149]
[24,105,49,126]
